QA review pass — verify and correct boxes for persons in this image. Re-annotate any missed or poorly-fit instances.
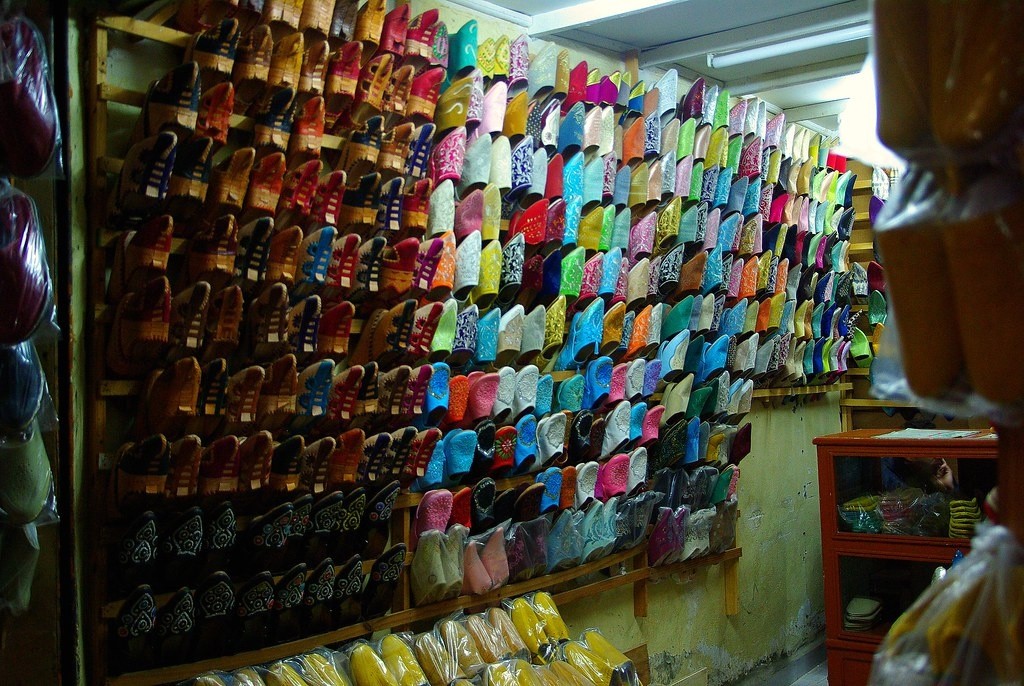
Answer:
[880,418,961,610]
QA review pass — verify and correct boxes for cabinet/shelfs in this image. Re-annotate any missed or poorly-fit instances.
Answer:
[812,427,1024,686]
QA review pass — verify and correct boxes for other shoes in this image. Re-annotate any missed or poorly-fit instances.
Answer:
[0,0,1024,686]
